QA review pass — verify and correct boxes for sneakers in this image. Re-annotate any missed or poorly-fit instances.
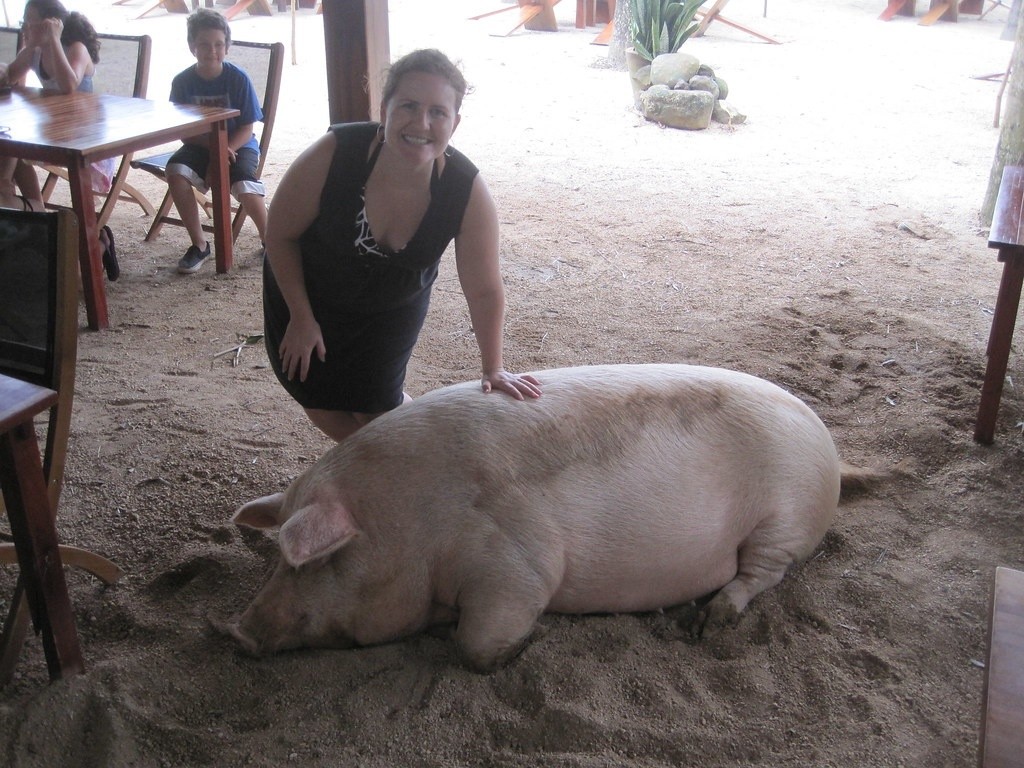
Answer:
[178,241,211,274]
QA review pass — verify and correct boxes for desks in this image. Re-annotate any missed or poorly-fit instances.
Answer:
[0,82,241,331]
[978,567,1024,768]
[0,374,100,679]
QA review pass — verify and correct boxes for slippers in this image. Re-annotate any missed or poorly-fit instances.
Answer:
[98,226,120,281]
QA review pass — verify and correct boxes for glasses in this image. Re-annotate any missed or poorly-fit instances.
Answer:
[19,21,41,28]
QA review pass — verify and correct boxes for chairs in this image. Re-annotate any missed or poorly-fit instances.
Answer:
[0,206,127,688]
[35,33,156,238]
[0,25,27,87]
[143,42,285,243]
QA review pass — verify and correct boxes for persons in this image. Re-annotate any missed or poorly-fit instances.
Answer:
[263,48,544,446]
[168,8,268,274]
[0,0,121,285]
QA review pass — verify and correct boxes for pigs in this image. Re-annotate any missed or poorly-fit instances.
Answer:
[223,362,889,672]
